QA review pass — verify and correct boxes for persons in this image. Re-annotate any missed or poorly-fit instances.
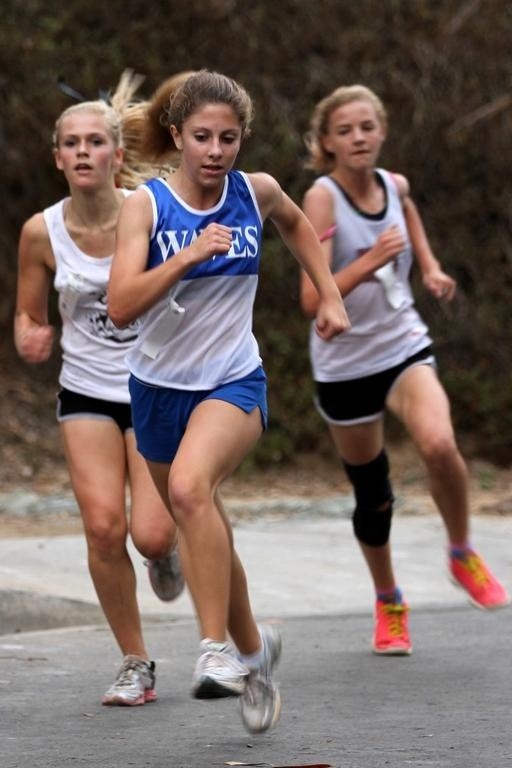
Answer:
[109,67,351,742]
[12,67,188,713]
[292,81,511,662]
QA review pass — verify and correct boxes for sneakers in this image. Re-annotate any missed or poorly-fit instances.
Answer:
[373,589,410,654]
[103,654,157,706]
[191,624,281,734]
[143,544,184,601]
[450,545,505,608]
